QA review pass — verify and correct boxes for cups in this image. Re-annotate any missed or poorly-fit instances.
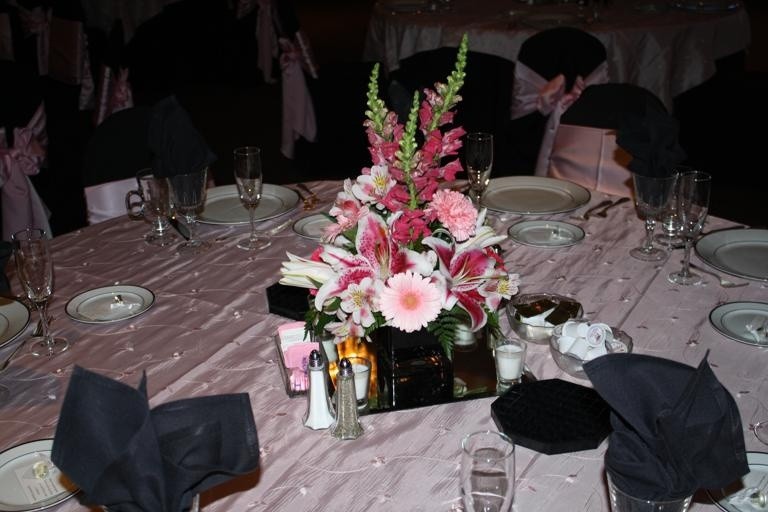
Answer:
[12,227,70,360]
[631,167,678,261]
[667,170,712,287]
[605,471,693,512]
[492,336,526,394]
[110,495,204,512]
[338,356,373,411]
[661,202,681,253]
[126,165,176,248]
[465,132,493,213]
[460,430,515,512]
[168,162,212,257]
[234,145,272,253]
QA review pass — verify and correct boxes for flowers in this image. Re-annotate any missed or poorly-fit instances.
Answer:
[278,32,520,368]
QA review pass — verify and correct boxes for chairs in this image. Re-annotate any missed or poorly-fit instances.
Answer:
[547,82,670,200]
[0,61,58,243]
[510,28,610,157]
[80,104,194,223]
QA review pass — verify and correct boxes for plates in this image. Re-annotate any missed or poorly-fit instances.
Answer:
[693,227,768,283]
[65,283,155,325]
[0,296,32,360]
[179,182,301,226]
[292,212,340,245]
[469,175,593,215]
[0,438,81,512]
[705,451,768,512]
[708,301,768,347]
[508,219,586,249]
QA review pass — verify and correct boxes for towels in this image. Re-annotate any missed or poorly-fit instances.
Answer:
[50,360,259,512]
[582,348,751,512]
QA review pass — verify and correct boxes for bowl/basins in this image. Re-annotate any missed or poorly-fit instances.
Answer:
[549,322,633,380]
[506,292,583,346]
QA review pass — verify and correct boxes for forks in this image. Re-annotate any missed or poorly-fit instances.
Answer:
[587,197,630,220]
[680,260,750,290]
[570,199,612,221]
[295,189,315,212]
[2,314,53,371]
[297,184,323,205]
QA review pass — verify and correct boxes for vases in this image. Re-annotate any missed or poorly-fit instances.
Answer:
[373,325,454,406]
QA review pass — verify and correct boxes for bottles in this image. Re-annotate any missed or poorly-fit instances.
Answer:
[329,357,364,440]
[300,350,336,431]
